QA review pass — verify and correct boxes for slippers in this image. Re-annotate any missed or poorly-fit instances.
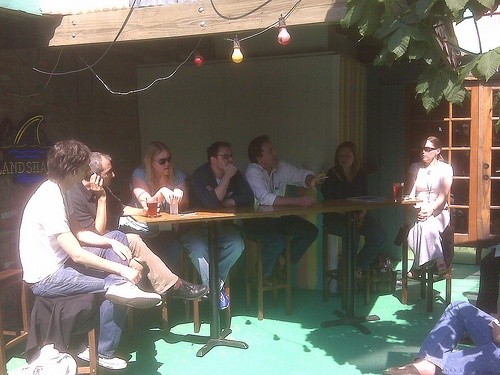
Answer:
[384,359,444,375]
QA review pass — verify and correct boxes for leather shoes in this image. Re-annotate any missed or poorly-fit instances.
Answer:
[167,279,207,301]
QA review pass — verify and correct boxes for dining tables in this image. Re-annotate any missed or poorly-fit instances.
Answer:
[130,198,423,357]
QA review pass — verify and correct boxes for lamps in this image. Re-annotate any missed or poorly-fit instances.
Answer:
[232,35,244,64]
[277,14,291,45]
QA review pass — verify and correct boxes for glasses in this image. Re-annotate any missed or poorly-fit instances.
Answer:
[418,147,438,152]
[153,156,171,164]
[217,153,233,159]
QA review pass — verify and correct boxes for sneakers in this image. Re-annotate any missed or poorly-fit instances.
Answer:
[75,342,128,370]
[105,280,162,309]
[218,285,229,309]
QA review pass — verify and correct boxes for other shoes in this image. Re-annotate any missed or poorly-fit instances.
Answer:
[436,264,447,275]
[408,268,423,280]
[265,256,288,287]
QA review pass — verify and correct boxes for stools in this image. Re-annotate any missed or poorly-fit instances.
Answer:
[115,220,454,334]
[0,268,31,375]
[36,295,97,375]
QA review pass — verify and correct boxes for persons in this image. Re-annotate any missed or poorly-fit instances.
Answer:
[179,141,255,310]
[19,140,162,370]
[476,244,500,320]
[234,136,329,285]
[119,141,189,274]
[321,141,386,281]
[384,301,500,375]
[65,152,208,299]
[401,135,454,280]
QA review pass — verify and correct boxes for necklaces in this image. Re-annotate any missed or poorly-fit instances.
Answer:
[424,162,438,204]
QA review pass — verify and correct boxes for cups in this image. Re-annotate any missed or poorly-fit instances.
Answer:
[254,197,262,209]
[146,197,158,218]
[170,199,178,216]
[393,182,404,200]
